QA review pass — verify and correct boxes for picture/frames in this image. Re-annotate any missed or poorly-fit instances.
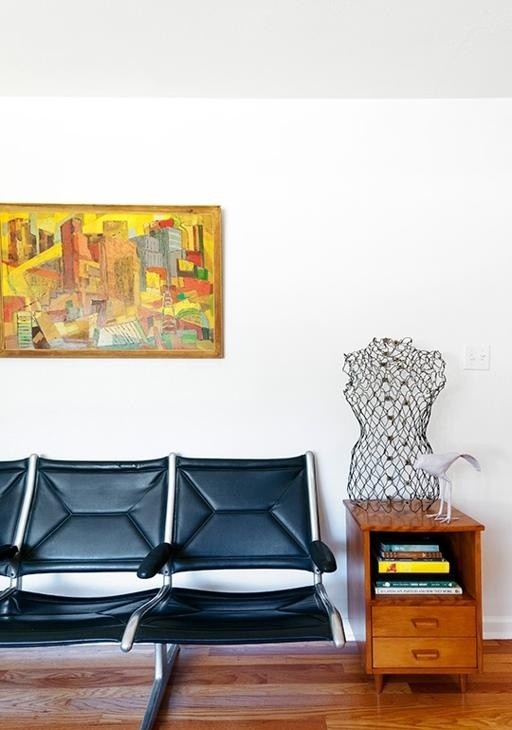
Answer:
[0,205,223,358]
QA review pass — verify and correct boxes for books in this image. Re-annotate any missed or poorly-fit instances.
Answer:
[374,542,463,596]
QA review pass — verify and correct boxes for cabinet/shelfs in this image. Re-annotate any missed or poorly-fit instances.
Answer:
[343,499,485,695]
